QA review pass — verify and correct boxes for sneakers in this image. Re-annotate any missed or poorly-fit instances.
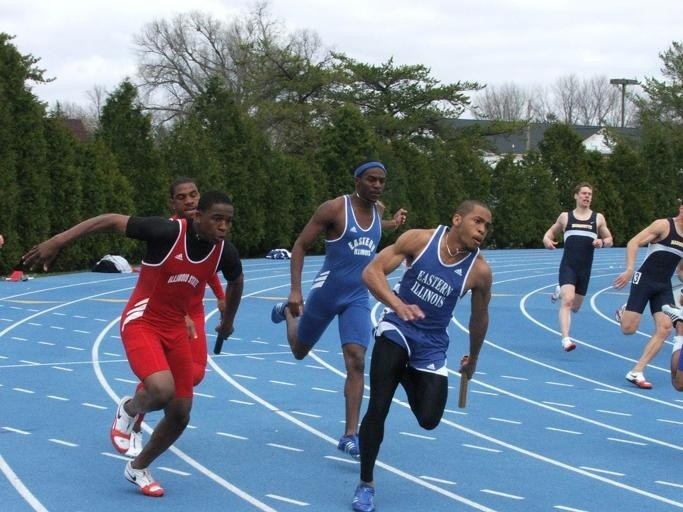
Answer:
[626,371,652,389]
[337,434,360,458]
[272,302,290,323]
[616,306,625,321]
[561,338,576,352]
[552,284,560,304]
[111,396,165,497]
[662,305,683,327]
[352,483,376,512]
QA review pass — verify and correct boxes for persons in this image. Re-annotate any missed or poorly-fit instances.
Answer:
[352,198,493,511]
[660,257,682,392]
[614,201,683,387]
[123,178,227,458]
[271,159,408,461]
[676,258,683,307]
[544,181,614,352]
[21,190,245,497]
[0,235,4,250]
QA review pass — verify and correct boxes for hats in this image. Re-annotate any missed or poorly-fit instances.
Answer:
[354,159,387,178]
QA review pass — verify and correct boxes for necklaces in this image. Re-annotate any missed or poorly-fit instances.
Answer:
[446,233,468,257]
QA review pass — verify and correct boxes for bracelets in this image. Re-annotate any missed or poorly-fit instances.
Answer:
[392,220,398,229]
[601,238,605,248]
